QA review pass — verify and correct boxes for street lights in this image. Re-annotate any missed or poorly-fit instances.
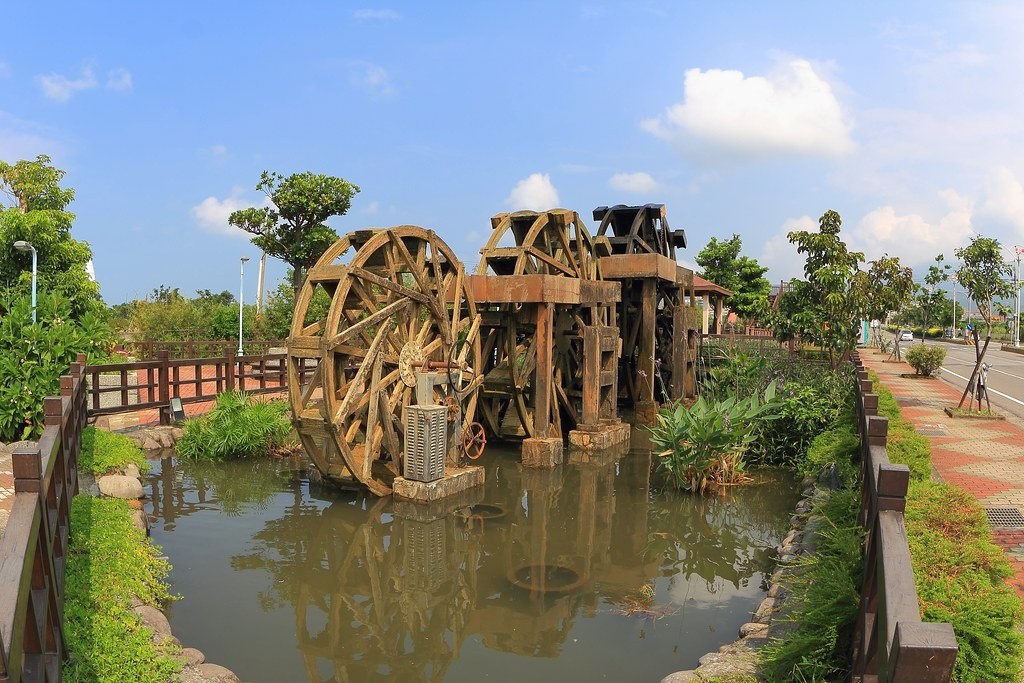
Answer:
[996,239,1020,347]
[236,257,249,367]
[14,240,37,326]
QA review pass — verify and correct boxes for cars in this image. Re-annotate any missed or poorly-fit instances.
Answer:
[899,329,914,341]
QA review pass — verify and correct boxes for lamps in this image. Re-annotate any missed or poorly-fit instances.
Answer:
[170,397,185,422]
